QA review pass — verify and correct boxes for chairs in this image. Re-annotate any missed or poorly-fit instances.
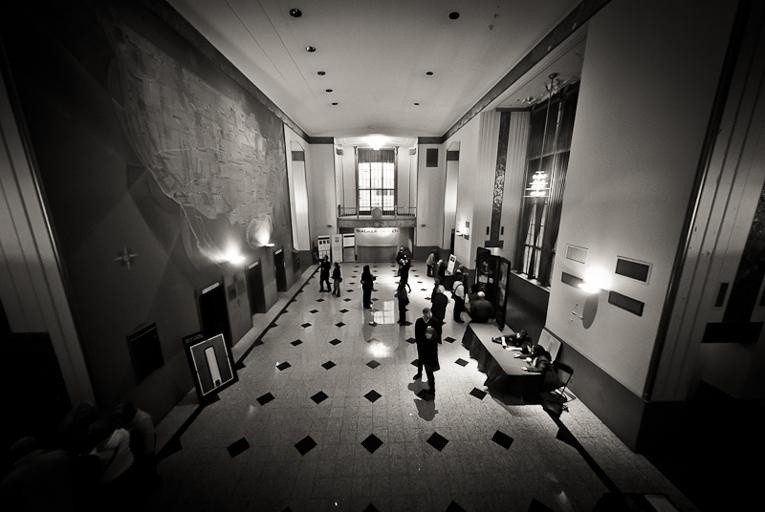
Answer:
[552,360,574,402]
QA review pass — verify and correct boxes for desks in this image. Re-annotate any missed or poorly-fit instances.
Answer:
[444,269,455,290]
[461,323,542,405]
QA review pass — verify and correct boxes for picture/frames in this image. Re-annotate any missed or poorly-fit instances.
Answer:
[185,330,239,402]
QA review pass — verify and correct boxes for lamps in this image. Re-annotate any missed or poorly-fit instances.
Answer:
[522,73,556,198]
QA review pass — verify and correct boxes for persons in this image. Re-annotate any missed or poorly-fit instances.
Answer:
[1,399,158,511]
[515,344,552,389]
[360,248,494,327]
[413,308,439,381]
[421,326,440,395]
[500,327,532,354]
[313,253,331,292]
[330,262,341,297]
